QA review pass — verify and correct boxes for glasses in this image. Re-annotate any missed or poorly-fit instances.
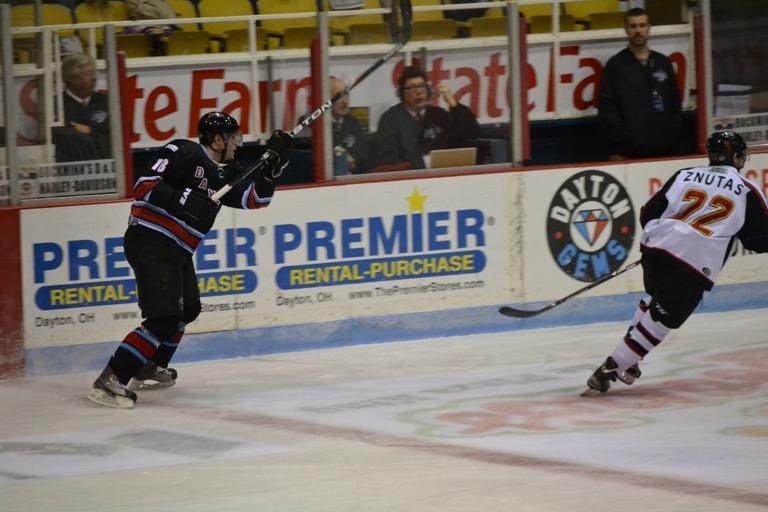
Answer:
[402,84,426,90]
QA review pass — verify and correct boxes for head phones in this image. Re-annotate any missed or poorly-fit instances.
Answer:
[396,68,434,100]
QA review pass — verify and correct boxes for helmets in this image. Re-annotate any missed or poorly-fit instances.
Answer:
[706,130,751,173]
[197,111,244,148]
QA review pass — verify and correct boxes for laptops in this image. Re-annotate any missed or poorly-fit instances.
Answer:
[430,147,478,168]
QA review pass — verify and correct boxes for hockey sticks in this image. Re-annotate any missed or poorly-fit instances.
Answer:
[499,261,644,317]
[209,0,413,199]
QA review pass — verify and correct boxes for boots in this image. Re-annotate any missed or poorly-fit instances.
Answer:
[92,355,138,403]
[134,360,178,382]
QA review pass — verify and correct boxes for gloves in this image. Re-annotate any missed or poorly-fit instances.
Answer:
[256,128,295,183]
[175,188,222,228]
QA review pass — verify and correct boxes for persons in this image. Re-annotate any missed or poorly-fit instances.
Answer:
[121,0,183,56]
[379,0,412,44]
[440,0,492,39]
[375,66,474,169]
[48,52,110,162]
[93,111,294,407]
[297,78,373,182]
[596,8,682,162]
[587,131,768,393]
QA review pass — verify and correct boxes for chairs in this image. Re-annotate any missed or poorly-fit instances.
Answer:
[2,1,627,61]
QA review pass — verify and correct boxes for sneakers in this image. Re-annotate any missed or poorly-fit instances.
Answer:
[586,355,620,393]
[624,363,643,378]
